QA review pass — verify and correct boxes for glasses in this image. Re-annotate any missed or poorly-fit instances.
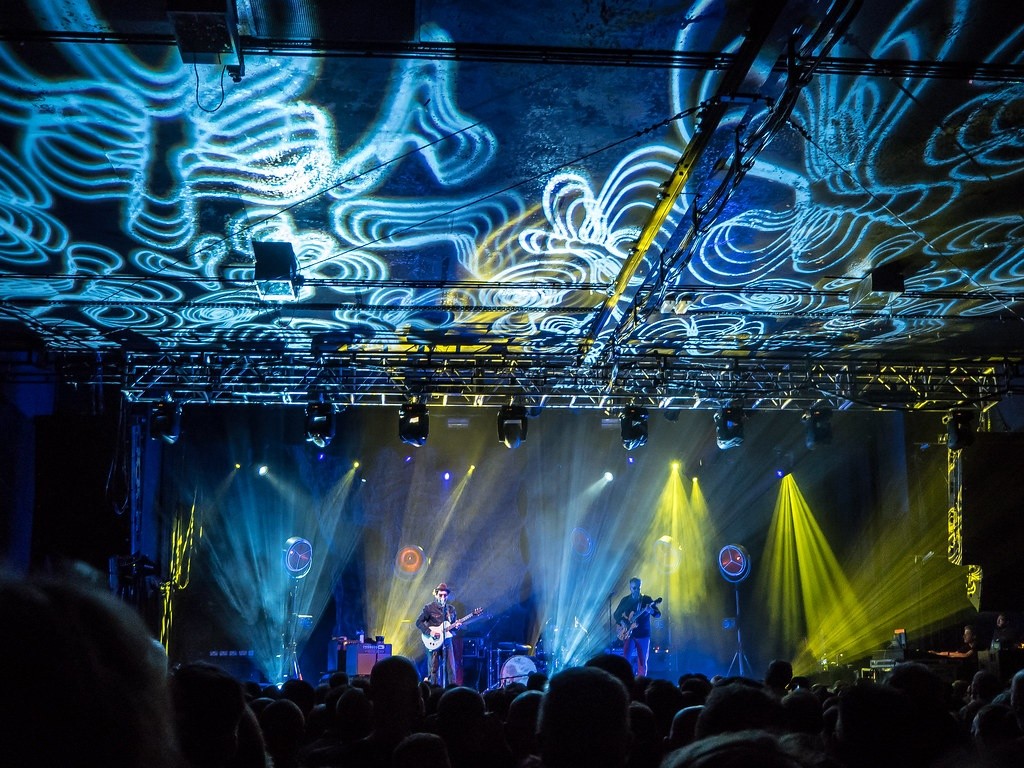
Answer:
[437,594,448,597]
[631,587,639,590]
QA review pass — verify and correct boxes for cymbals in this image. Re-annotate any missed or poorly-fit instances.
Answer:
[498,642,532,651]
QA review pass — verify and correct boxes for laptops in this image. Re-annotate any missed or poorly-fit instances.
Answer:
[893,628,911,651]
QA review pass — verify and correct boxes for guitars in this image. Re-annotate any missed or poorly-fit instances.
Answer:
[421,607,483,651]
[615,596,663,641]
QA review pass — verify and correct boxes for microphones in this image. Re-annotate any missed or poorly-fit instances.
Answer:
[546,618,552,624]
[528,671,534,676]
[441,598,446,606]
[575,616,578,627]
[607,592,615,599]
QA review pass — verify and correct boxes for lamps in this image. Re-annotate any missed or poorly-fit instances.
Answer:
[621,407,650,453]
[304,404,340,448]
[715,408,747,450]
[497,406,527,449]
[150,398,185,444]
[949,411,977,450]
[397,403,430,449]
[801,410,835,451]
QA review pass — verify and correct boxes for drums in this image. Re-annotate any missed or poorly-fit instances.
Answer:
[500,655,541,689]
[537,652,552,661]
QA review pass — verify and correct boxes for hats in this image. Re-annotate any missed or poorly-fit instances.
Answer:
[433,583,451,595]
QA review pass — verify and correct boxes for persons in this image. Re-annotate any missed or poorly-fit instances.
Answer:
[614,578,661,676]
[1,578,1024,768]
[416,583,462,684]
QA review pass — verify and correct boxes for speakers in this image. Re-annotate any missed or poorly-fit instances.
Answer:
[345,643,392,676]
